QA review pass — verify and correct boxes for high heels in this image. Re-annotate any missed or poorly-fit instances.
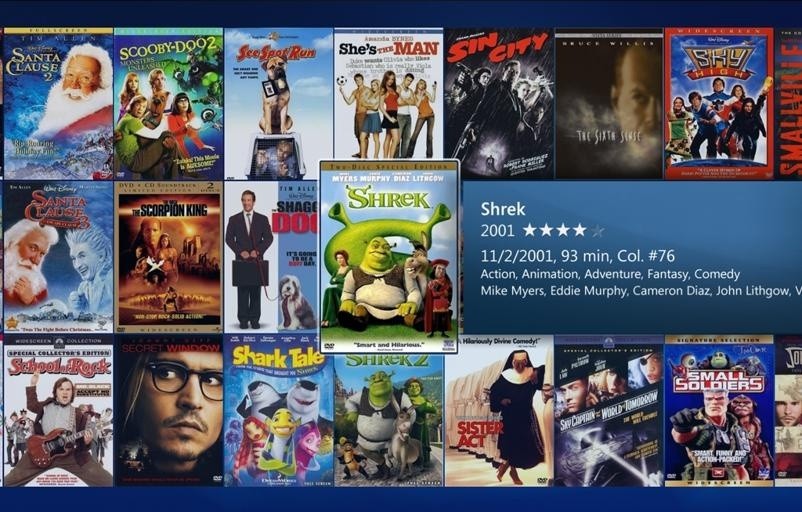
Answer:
[510,469,523,484]
[497,464,507,482]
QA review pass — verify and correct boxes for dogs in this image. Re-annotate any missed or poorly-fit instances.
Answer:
[277,275,317,330]
[259,53,294,135]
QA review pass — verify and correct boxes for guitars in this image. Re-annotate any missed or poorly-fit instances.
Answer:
[28,420,112,468]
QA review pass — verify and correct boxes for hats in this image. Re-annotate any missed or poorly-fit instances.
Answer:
[513,352,526,361]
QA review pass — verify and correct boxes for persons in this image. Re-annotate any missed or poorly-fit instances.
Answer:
[118,349,224,487]
[608,42,662,172]
[586,370,627,401]
[446,61,553,180]
[115,68,217,180]
[665,73,774,163]
[336,69,438,160]
[63,223,113,318]
[129,214,184,314]
[774,402,802,425]
[555,380,587,420]
[32,43,113,143]
[256,141,298,178]
[5,218,60,309]
[635,349,661,387]
[3,362,112,487]
[225,189,275,329]
[486,348,552,487]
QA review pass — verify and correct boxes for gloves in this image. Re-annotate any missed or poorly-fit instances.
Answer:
[670,407,706,434]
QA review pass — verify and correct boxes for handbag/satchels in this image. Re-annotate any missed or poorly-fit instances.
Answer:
[232,257,268,286]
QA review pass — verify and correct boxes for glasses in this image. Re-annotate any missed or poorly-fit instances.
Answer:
[144,359,223,402]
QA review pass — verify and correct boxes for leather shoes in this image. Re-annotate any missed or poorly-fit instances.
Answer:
[240,320,248,328]
[250,320,260,328]
